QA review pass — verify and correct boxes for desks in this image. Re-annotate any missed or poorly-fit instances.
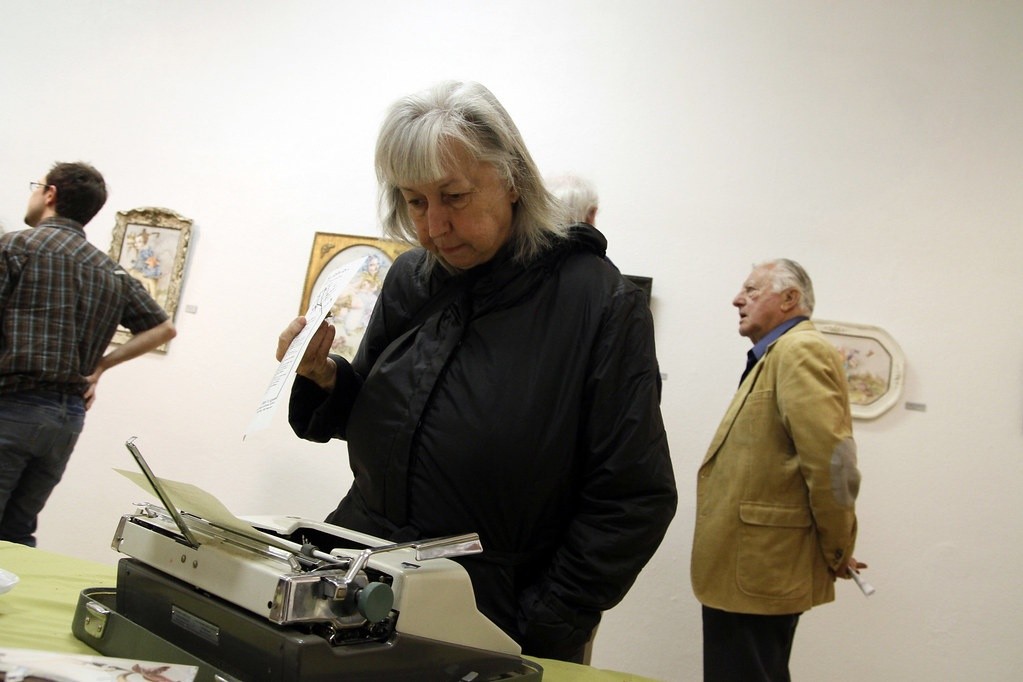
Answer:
[0,538,665,682]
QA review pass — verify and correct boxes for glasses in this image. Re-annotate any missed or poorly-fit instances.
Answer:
[30,182,50,194]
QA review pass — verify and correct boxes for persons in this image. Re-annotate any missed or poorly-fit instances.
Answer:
[276,79,678,681]
[691,259,868,682]
[0,158,177,548]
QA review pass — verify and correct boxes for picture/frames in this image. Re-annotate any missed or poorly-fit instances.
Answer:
[812,318,905,419]
[298,232,422,364]
[107,208,193,353]
